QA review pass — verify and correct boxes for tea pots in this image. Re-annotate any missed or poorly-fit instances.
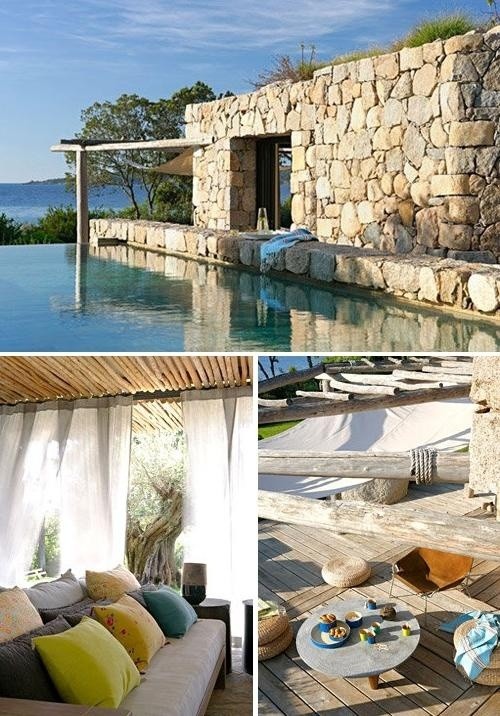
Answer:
[380,605,397,620]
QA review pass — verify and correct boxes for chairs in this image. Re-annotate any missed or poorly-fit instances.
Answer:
[389,535,472,627]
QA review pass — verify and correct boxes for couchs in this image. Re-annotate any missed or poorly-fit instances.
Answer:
[0,563,226,715]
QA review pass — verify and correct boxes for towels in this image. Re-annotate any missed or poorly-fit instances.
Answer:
[259,228,317,263]
[436,609,499,682]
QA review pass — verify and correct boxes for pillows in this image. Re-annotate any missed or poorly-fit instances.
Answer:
[28,614,143,708]
[23,567,85,609]
[0,585,42,642]
[142,576,199,639]
[90,592,167,672]
[128,584,147,605]
[80,563,138,601]
[0,615,71,701]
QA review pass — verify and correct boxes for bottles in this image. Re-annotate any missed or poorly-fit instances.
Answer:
[257,207,269,231]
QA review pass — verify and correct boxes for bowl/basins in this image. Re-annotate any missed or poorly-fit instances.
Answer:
[309,620,351,650]
[329,627,346,641]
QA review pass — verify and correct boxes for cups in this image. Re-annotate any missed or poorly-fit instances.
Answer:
[344,611,362,628]
[402,628,411,637]
[365,599,376,609]
[319,620,336,632]
[359,622,381,644]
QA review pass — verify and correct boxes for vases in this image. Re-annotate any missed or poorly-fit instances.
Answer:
[256,206,268,230]
[182,562,207,603]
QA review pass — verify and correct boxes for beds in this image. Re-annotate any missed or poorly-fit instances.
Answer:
[197,598,232,677]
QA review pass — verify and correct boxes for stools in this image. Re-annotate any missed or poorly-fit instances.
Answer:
[258,606,293,659]
[453,620,499,688]
